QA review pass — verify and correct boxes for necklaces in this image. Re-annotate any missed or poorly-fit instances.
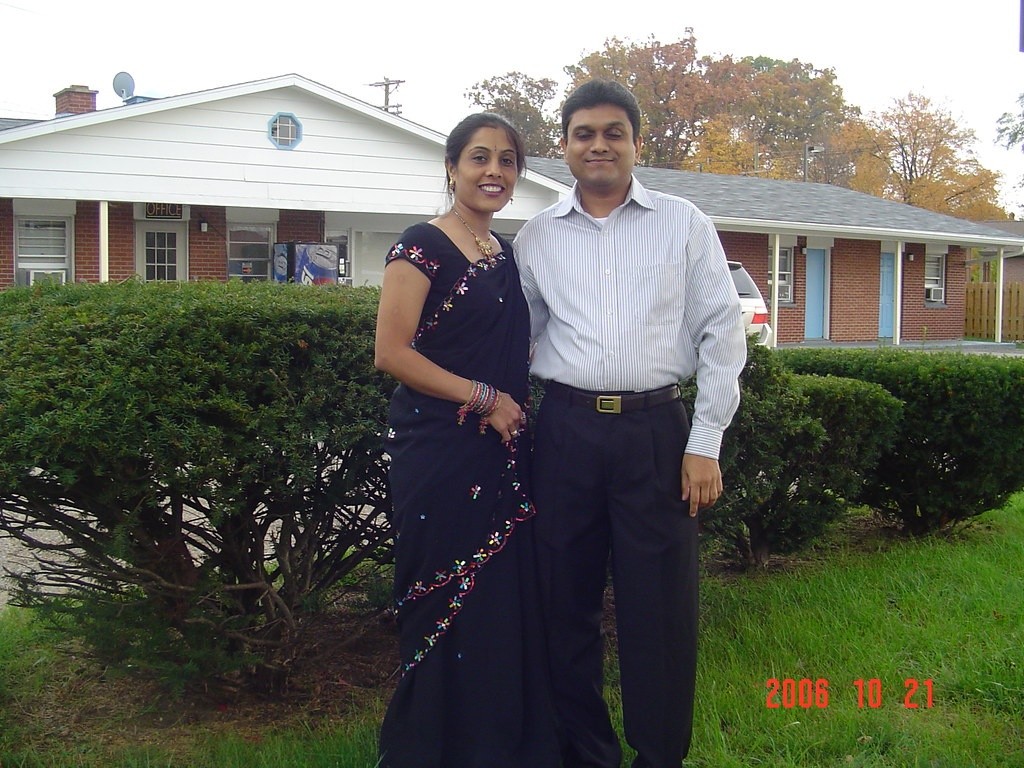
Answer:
[451,208,493,255]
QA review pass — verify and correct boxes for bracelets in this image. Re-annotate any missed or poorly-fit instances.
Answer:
[456,380,500,435]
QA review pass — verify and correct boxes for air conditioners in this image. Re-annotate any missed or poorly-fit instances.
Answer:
[767,284,792,301]
[25,269,66,287]
[925,286,946,302]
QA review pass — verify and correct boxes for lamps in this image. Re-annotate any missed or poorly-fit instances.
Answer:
[801,244,807,256]
[200,215,209,233]
[907,251,914,263]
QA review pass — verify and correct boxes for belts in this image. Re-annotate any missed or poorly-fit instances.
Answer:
[544,380,680,414]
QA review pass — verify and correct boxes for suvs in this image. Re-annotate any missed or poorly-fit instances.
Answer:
[727,260,774,351]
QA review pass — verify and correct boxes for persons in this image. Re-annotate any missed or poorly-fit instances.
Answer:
[242,261,252,275]
[510,79,748,767]
[375,113,534,768]
